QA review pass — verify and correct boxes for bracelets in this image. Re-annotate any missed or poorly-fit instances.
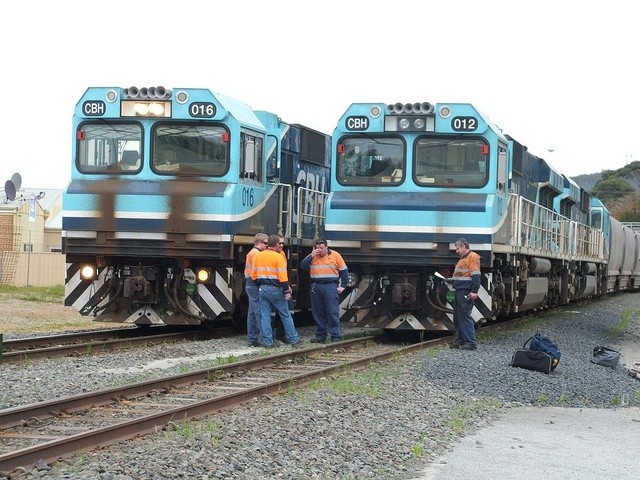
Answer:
[284,289,291,294]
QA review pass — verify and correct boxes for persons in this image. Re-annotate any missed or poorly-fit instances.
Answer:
[446,238,480,350]
[300,239,349,343]
[251,234,304,347]
[247,233,269,347]
[275,234,291,344]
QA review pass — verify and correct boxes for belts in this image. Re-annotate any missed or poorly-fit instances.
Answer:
[311,280,337,285]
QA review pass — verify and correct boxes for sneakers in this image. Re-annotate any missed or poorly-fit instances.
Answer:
[247,340,263,347]
[265,341,281,349]
[331,338,342,342]
[289,338,305,347]
[449,339,463,349]
[275,333,288,343]
[311,336,325,344]
[459,343,477,351]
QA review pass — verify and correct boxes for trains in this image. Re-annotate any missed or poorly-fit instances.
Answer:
[323,103,639,334]
[62,87,332,330]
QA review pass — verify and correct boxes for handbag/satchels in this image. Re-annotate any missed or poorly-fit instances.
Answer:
[522,334,561,370]
[511,348,552,375]
[590,345,621,370]
[628,362,640,380]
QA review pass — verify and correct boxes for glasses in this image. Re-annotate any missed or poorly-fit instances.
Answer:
[315,239,325,243]
[279,242,284,245]
[261,241,267,245]
